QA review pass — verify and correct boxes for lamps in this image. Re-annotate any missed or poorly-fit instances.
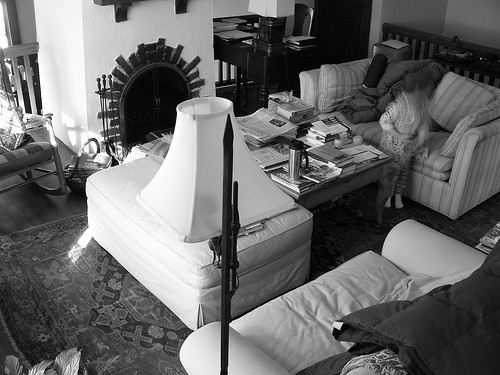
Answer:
[135,96,294,375]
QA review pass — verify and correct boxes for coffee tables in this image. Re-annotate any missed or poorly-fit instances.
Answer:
[121,99,395,234]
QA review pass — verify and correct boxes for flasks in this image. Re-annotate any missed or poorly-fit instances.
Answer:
[288,139,308,180]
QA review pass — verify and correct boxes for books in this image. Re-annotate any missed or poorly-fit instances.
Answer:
[268,91,315,124]
[215,16,271,52]
[282,36,318,51]
[65,152,113,184]
[475,222,500,254]
[238,223,263,236]
[235,107,298,148]
[139,139,170,159]
[250,113,389,193]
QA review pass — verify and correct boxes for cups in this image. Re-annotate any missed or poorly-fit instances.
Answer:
[353,135,364,144]
[334,139,345,148]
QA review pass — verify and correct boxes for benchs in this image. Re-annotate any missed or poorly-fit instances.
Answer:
[84,154,314,331]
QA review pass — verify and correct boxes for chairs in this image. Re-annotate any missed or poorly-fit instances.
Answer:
[0,46,68,196]
[276,3,319,94]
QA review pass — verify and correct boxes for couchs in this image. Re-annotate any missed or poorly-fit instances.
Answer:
[299,54,500,221]
[178,219,500,375]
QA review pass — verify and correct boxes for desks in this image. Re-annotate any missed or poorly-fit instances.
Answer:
[213,14,327,109]
[425,46,500,80]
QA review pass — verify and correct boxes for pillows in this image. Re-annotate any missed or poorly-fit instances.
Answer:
[426,72,494,133]
[318,58,373,113]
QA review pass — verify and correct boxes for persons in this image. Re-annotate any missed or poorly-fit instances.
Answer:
[379,71,431,208]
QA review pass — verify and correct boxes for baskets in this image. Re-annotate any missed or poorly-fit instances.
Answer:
[63,138,113,192]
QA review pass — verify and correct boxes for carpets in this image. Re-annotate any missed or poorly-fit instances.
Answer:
[0,195,500,375]
